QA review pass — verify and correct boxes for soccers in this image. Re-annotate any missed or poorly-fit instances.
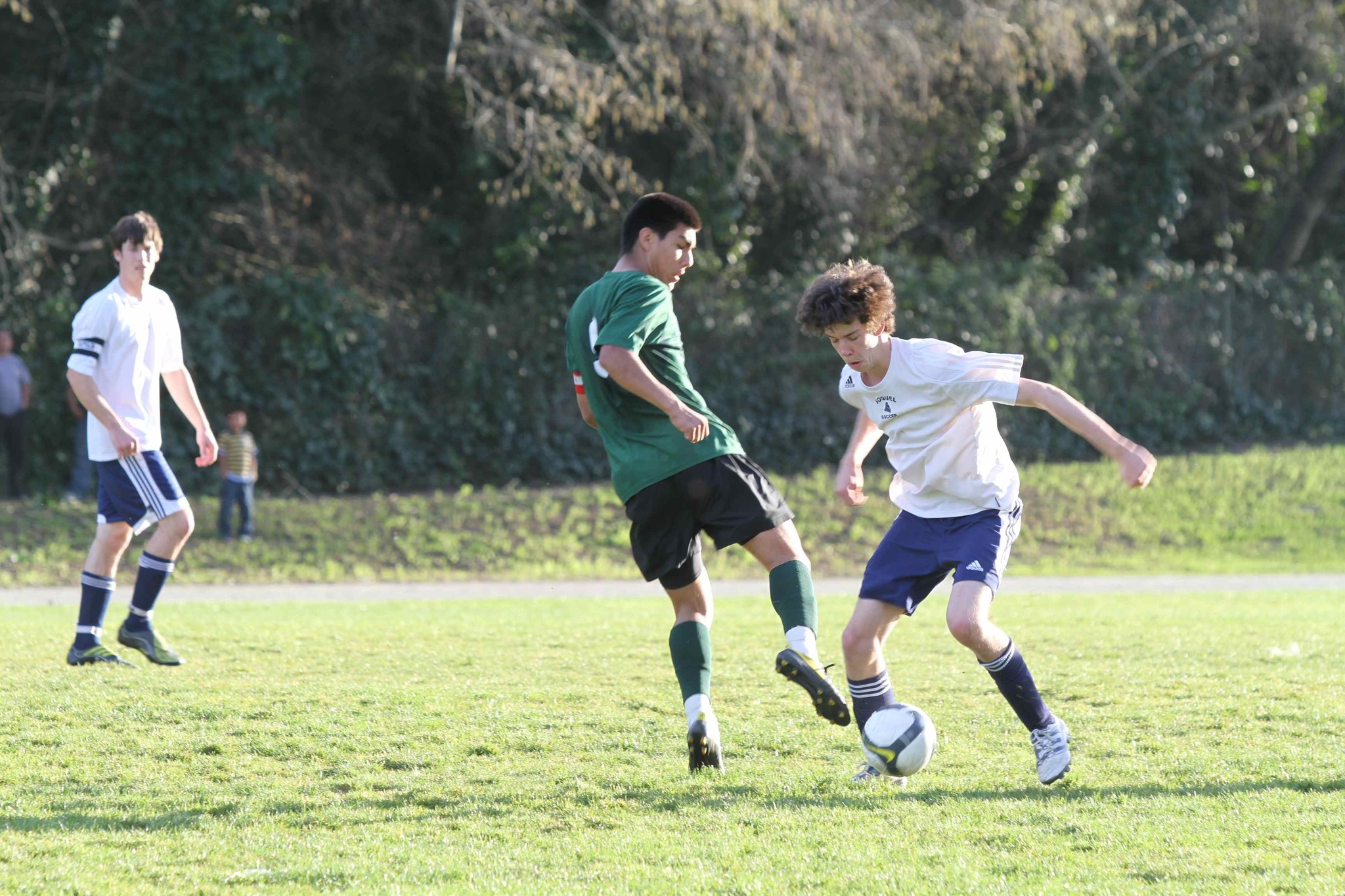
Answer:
[858,703,938,778]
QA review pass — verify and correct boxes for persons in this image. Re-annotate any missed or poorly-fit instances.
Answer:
[795,258,1158,786]
[564,193,851,778]
[65,380,96,504]
[215,407,259,543]
[64,210,218,668]
[0,330,33,500]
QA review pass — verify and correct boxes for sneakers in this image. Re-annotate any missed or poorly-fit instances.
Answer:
[776,649,851,727]
[117,621,185,665]
[67,645,141,670]
[686,717,724,776]
[1031,715,1072,785]
[848,765,908,787]
